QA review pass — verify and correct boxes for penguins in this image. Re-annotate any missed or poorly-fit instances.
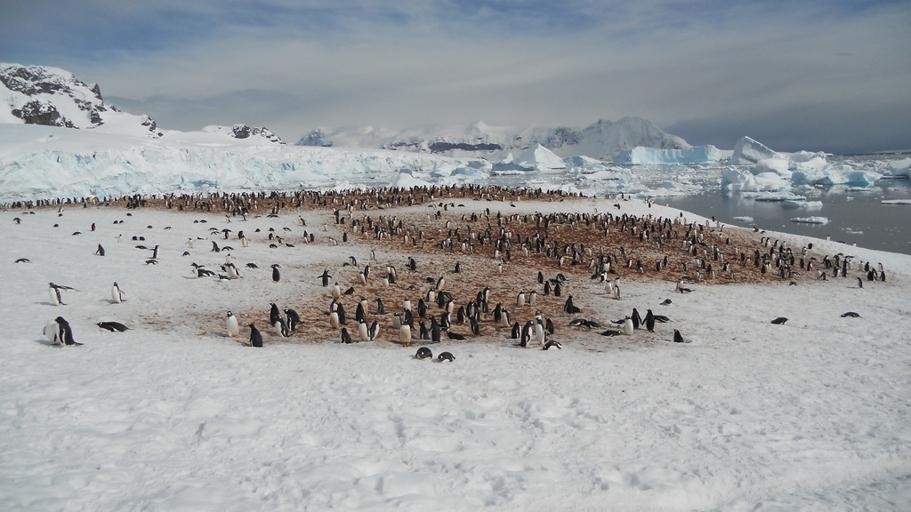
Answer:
[1,182,888,351]
[416,347,433,360]
[437,352,455,363]
